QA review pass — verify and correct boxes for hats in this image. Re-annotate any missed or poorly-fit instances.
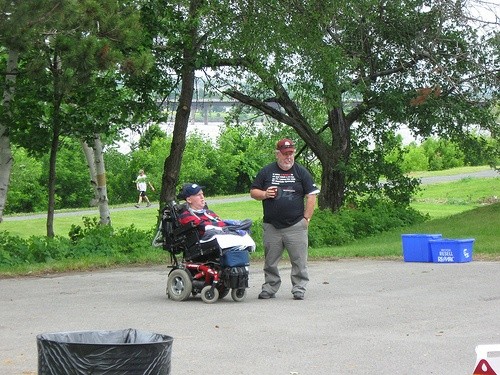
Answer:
[183,184,206,200]
[277,138,295,154]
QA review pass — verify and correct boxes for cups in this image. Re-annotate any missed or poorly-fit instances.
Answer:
[268,186,277,197]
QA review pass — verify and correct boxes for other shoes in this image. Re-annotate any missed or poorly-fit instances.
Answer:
[146,202,151,207]
[293,292,304,300]
[135,204,140,208]
[258,291,275,299]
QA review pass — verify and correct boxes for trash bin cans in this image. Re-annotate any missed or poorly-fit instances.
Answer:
[37,329,173,375]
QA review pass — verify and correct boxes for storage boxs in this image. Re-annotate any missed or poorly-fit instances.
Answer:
[429,237,475,264]
[401,233,442,263]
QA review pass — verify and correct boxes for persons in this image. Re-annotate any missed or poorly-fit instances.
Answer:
[175,183,256,281]
[132,170,155,208]
[250,137,321,300]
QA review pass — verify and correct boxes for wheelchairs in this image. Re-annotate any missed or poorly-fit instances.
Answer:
[156,196,251,304]
